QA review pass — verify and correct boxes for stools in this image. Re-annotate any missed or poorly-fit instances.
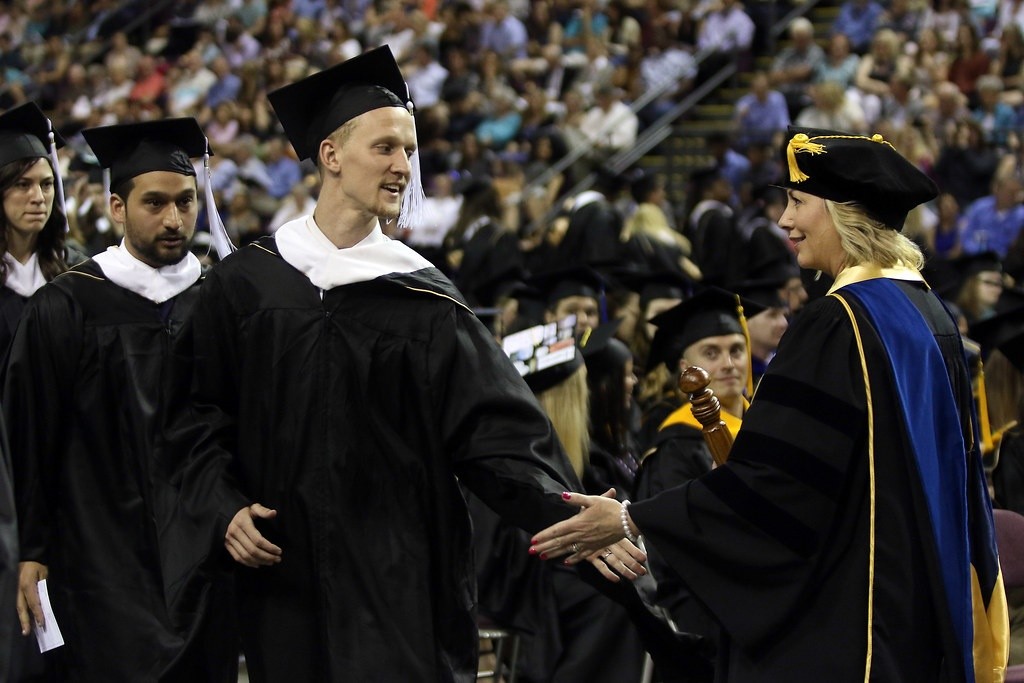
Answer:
[477,614,520,683]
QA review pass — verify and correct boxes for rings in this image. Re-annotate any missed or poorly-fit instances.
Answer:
[604,551,611,559]
[571,543,581,552]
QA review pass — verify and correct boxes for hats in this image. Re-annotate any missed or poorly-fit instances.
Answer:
[948,250,1002,276]
[768,124,942,231]
[0,101,70,233]
[536,264,623,324]
[577,317,631,376]
[267,44,438,230]
[499,314,584,393]
[81,117,236,261]
[967,305,1024,371]
[645,286,767,398]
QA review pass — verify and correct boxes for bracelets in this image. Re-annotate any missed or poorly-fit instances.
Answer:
[620,500,641,543]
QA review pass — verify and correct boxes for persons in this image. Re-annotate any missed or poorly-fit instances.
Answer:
[0,0,1024,683]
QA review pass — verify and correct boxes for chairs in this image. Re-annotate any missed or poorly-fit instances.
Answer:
[991,508,1024,683]
